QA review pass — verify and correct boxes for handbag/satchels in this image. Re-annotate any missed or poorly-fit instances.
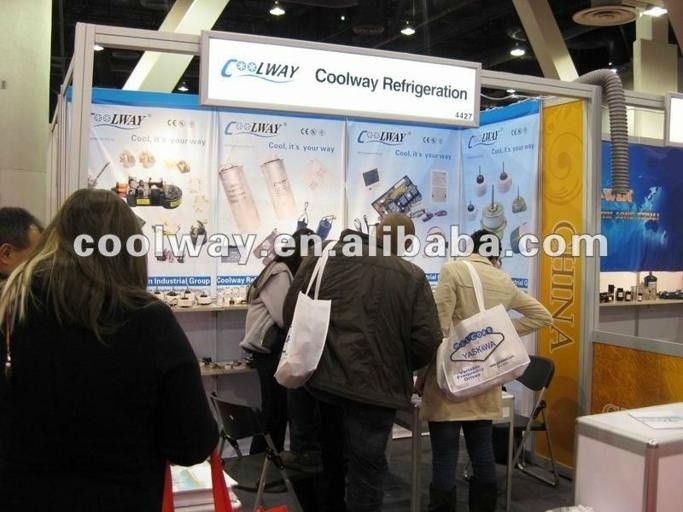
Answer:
[273,239,338,389]
[434,261,531,403]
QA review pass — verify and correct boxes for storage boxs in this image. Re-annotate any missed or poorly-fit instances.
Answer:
[574,403,683,512]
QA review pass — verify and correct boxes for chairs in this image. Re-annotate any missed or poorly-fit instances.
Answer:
[210,390,305,512]
[461,354,561,490]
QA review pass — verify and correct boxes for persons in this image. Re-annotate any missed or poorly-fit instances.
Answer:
[0,206,47,284]
[1,186,221,512]
[281,211,445,509]
[239,227,318,493]
[414,230,555,511]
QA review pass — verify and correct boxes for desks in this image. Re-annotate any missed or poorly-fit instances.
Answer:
[384,376,515,511]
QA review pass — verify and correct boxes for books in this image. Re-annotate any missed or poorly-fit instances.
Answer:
[169,461,242,512]
[628,410,683,429]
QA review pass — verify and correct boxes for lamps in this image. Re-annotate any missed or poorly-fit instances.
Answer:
[508,26,528,56]
[177,80,189,92]
[269,0,285,15]
[400,17,415,35]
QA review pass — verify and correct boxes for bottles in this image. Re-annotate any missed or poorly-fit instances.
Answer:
[607,271,657,302]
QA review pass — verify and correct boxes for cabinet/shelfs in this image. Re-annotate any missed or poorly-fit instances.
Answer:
[152,289,264,459]
